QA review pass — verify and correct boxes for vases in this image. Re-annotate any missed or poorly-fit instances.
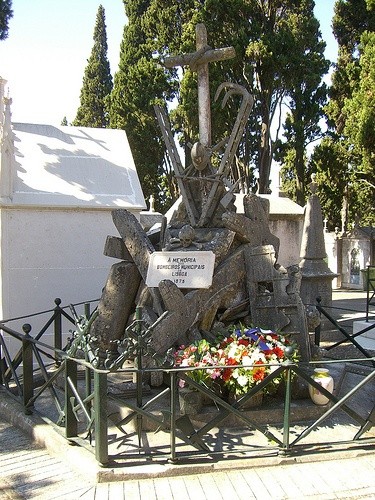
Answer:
[178,387,201,412]
[308,368,334,405]
[228,387,263,409]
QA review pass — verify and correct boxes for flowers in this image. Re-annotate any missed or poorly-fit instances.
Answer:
[172,338,225,392]
[217,320,301,394]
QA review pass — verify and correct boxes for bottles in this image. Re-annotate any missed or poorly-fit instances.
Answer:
[309,368,334,405]
